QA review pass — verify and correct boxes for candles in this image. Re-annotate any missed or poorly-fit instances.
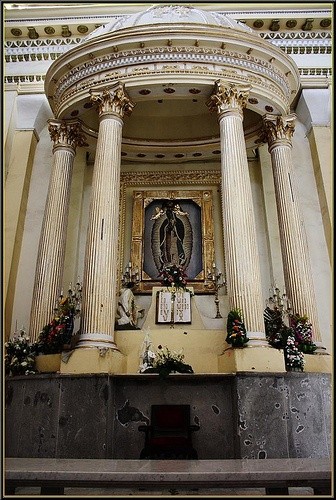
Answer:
[204,263,226,285]
[54,274,82,309]
[122,261,141,282]
[269,284,291,311]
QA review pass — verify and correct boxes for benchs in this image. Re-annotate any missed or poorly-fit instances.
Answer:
[5,458,332,496]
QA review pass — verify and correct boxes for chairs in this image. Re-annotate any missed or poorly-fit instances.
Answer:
[138,404,200,459]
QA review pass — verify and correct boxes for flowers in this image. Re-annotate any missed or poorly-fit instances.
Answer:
[140,343,193,374]
[263,305,327,372]
[225,309,250,347]
[4,297,79,376]
[158,265,187,289]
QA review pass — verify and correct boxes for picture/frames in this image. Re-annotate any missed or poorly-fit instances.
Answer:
[131,188,216,294]
